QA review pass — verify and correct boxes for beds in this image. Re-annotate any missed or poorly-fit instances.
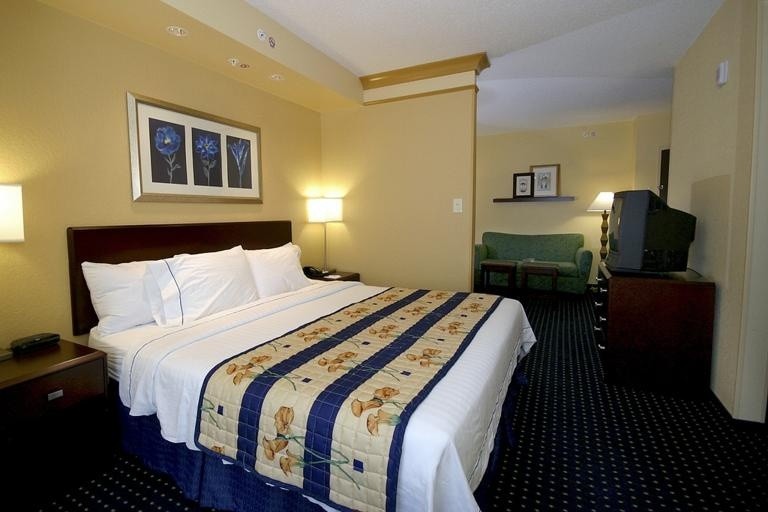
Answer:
[66,220,538,512]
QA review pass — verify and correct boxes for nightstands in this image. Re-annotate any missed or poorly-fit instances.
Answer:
[307,272,360,281]
[1,339,108,487]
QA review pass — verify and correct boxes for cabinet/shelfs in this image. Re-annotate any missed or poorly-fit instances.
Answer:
[594,262,715,399]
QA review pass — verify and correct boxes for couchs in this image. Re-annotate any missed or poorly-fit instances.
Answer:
[475,232,593,295]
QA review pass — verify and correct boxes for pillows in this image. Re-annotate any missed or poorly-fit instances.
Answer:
[81,260,157,335]
[246,241,309,299]
[145,245,258,328]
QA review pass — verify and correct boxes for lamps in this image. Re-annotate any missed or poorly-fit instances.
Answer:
[307,196,344,274]
[0,182,26,245]
[587,192,614,260]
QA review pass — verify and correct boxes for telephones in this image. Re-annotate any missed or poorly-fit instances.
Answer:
[303,267,329,277]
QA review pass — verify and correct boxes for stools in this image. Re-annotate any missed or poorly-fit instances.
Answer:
[521,262,558,293]
[479,261,517,292]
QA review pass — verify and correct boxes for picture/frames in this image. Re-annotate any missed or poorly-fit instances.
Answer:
[513,172,534,197]
[530,163,560,198]
[126,90,264,205]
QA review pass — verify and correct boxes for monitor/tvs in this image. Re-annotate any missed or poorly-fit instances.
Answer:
[605,189,697,277]
